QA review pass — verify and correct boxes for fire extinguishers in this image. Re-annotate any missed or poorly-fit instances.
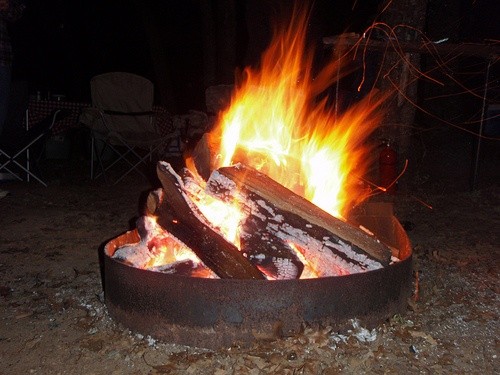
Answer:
[376,136,399,196]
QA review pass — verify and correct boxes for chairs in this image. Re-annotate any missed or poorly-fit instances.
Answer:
[75,71,184,191]
[0,100,73,188]
